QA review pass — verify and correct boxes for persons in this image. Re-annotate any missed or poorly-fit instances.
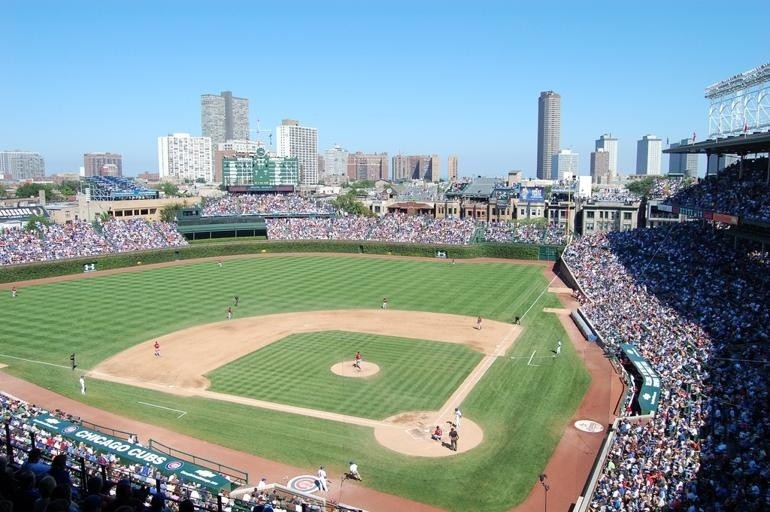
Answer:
[1,395,361,511]
[153,341,161,359]
[355,351,362,370]
[234,296,240,307]
[449,428,459,451]
[70,352,78,371]
[454,408,462,428]
[80,376,86,395]
[549,177,577,205]
[514,316,522,325]
[477,316,482,330]
[1,217,189,267]
[401,182,519,201]
[555,338,562,356]
[265,212,566,246]
[564,156,770,512]
[382,297,387,309]
[432,426,442,441]
[227,307,232,320]
[12,286,16,298]
[200,192,348,218]
